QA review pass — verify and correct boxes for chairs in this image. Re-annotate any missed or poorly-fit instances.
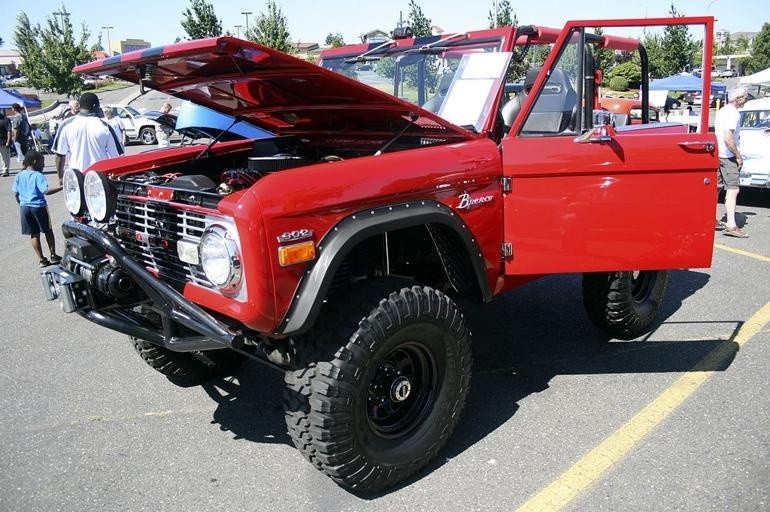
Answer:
[501,68,581,140]
[418,71,454,113]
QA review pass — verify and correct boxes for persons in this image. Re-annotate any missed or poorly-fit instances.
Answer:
[155,103,173,148]
[714,86,748,238]
[12,152,63,269]
[51,93,125,191]
[100,105,126,154]
[20,106,27,121]
[62,100,80,121]
[12,103,28,165]
[0,112,12,177]
[686,106,696,133]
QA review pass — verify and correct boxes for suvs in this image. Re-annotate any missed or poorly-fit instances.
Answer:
[40,13,722,503]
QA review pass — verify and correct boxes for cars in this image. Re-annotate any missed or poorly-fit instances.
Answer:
[354,65,373,72]
[0,69,173,146]
[599,65,770,194]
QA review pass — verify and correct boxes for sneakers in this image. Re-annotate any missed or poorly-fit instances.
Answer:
[715,220,727,228]
[51,254,62,261]
[722,226,748,237]
[39,258,50,267]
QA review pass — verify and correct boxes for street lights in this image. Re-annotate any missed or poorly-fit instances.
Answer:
[100,26,115,57]
[241,12,252,39]
[52,12,70,44]
[234,25,243,39]
